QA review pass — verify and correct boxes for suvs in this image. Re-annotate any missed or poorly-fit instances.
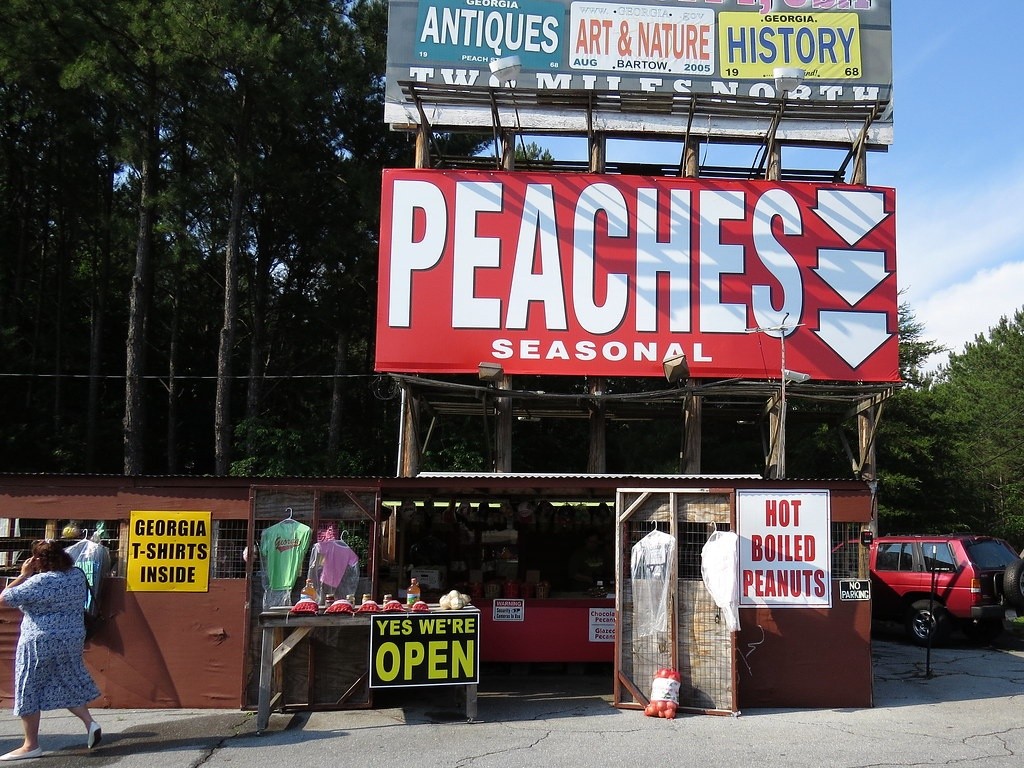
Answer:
[832,534,1024,638]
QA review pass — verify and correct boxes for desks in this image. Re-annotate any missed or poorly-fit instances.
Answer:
[257,603,479,734]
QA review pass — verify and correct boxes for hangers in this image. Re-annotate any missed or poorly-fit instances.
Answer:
[77,529,98,550]
[279,507,297,524]
[633,520,675,554]
[333,530,350,548]
[702,521,736,552]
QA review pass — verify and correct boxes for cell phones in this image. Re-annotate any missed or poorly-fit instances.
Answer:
[32,558,41,569]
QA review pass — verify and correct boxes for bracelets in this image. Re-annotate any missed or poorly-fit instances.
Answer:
[18,574,27,579]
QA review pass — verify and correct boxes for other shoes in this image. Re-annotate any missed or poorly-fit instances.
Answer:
[0,745,42,761]
[88,722,102,748]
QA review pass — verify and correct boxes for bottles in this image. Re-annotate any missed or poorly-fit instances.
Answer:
[407,578,420,608]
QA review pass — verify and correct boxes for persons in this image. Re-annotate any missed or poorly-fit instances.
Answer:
[565,533,609,590]
[0,537,102,763]
